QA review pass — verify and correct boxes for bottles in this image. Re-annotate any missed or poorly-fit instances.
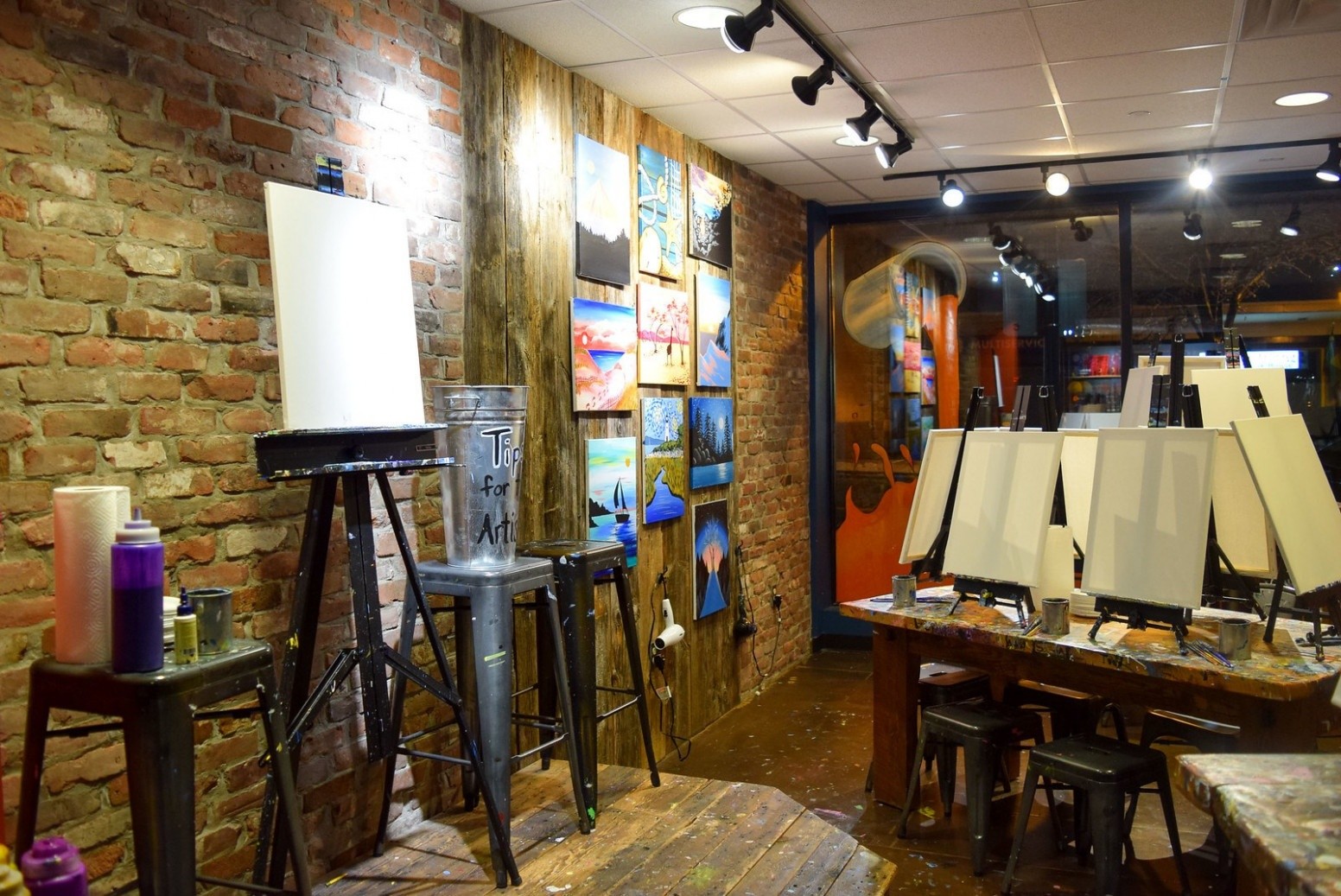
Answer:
[0,845,31,896]
[174,586,198,665]
[110,507,162,673]
[22,836,89,896]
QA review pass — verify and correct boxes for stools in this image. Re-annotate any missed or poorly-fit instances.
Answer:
[867,663,1241,896]
[377,538,658,887]
[14,639,312,896]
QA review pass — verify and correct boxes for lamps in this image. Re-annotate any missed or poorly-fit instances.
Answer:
[847,106,882,142]
[1316,143,1341,182]
[876,131,912,169]
[1070,218,1093,241]
[791,60,836,106]
[1040,166,1070,196]
[1187,153,1212,189]
[720,5,774,53]
[988,222,1057,302]
[1281,201,1302,236]
[936,174,965,208]
[1183,208,1202,241]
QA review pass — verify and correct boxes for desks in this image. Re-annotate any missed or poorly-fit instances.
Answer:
[1176,754,1341,896]
[840,584,1341,896]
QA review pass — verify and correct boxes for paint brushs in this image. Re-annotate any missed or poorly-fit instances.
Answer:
[1185,638,1235,668]
[870,597,954,603]
[1023,615,1042,636]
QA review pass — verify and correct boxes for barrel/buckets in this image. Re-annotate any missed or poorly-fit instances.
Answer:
[891,575,916,607]
[1041,598,1070,634]
[430,383,531,572]
[187,588,234,655]
[1218,617,1251,661]
